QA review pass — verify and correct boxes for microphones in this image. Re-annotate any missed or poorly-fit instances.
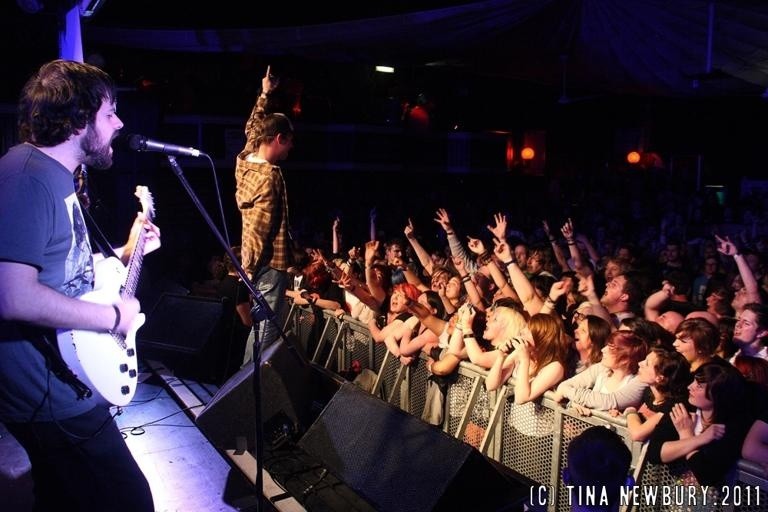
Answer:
[129,133,203,160]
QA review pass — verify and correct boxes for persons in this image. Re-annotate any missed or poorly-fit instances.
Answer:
[189,208,768,465]
[0,60,163,510]
[562,427,637,512]
[234,65,294,370]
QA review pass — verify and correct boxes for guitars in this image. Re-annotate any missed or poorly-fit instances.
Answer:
[56,184,155,407]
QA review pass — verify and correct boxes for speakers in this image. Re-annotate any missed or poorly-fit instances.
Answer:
[137,291,226,363]
[295,382,502,512]
[195,329,314,450]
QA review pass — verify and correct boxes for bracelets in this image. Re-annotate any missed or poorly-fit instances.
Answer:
[110,304,121,335]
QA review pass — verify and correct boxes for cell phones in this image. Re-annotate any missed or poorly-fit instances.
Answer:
[467,304,473,310]
